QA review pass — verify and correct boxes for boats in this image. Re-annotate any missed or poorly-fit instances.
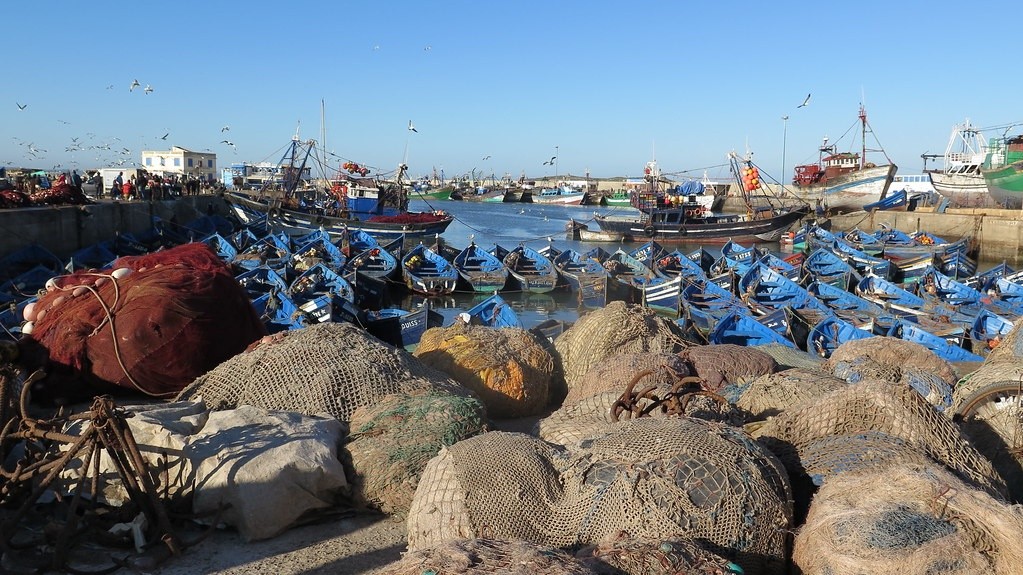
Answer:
[202,101,1023,362]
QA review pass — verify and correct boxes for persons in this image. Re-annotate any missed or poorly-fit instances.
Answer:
[110,172,207,202]
[25,170,82,195]
[94,172,102,199]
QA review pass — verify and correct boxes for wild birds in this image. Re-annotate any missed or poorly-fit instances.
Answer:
[482,156,491,160]
[408,119,418,133]
[2,80,236,168]
[797,94,811,108]
[543,156,556,166]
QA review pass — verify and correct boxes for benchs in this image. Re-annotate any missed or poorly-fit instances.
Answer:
[723,330,763,337]
[618,266,643,275]
[978,333,1007,340]
[811,261,843,272]
[690,294,725,305]
[756,282,805,305]
[885,238,910,243]
[463,257,498,268]
[312,291,329,295]
[666,269,680,273]
[941,297,973,301]
[413,268,442,275]
[999,293,1020,297]
[247,281,276,294]
[367,257,392,267]
[516,259,549,273]
[816,295,874,316]
[562,262,594,270]
[877,294,900,298]
[938,289,957,292]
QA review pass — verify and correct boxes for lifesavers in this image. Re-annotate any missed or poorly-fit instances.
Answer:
[684,210,692,217]
[267,207,280,220]
[679,226,686,235]
[643,226,655,237]
[315,215,325,224]
[694,208,701,215]
